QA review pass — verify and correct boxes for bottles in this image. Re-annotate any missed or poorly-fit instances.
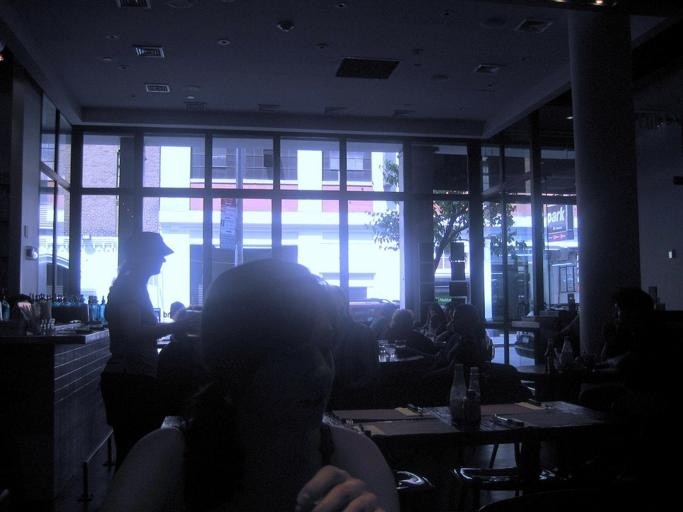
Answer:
[378,344,387,362]
[450,363,482,430]
[0,296,11,320]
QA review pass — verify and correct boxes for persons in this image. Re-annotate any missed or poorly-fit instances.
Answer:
[162,285,682,480]
[98,229,204,476]
[97,256,399,511]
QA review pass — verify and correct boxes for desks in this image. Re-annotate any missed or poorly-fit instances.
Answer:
[321,400,610,467]
[375,337,424,363]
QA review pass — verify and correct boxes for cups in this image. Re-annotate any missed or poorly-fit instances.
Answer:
[387,347,397,359]
[87,294,107,321]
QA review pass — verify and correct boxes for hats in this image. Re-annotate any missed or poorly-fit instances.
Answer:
[127,232,173,256]
